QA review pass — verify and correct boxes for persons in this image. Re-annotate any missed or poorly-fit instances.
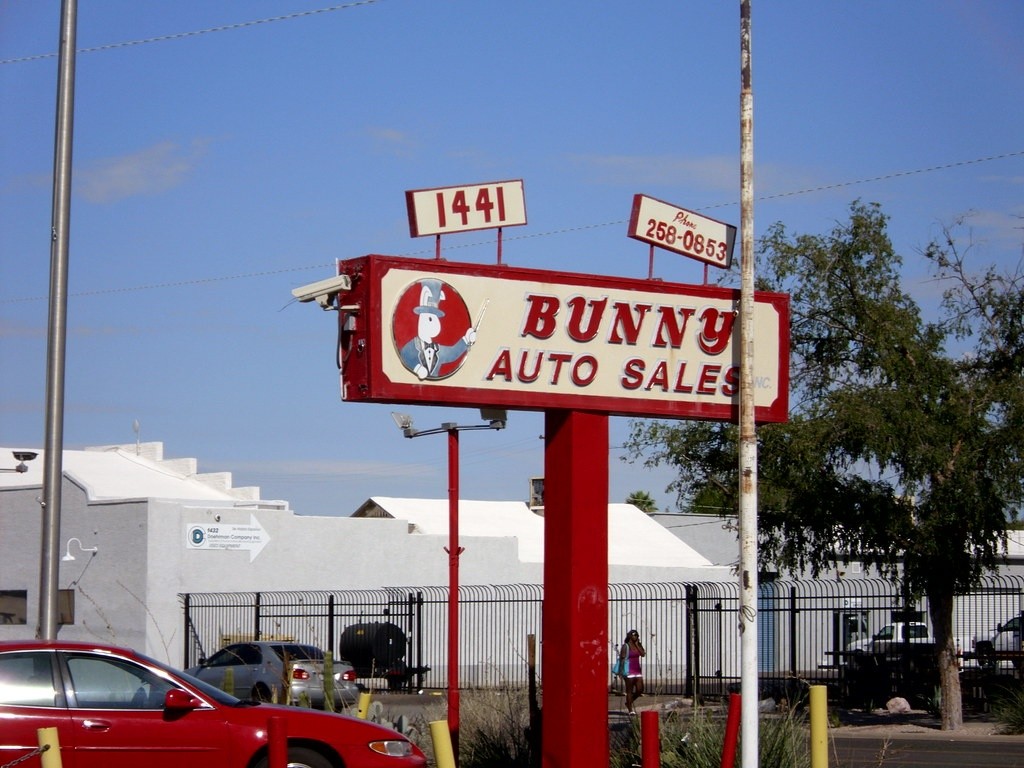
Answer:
[619,630,646,715]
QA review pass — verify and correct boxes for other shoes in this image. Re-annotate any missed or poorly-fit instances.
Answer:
[629,712,636,715]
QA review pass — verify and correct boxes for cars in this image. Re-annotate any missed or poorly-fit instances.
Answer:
[182,641,359,714]
[847,615,1024,685]
[0,638,428,768]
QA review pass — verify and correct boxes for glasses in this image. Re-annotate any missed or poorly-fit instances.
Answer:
[632,634,639,637]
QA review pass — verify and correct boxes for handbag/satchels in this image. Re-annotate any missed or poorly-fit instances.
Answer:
[611,643,629,676]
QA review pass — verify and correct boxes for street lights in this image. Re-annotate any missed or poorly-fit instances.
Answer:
[392,407,510,766]
